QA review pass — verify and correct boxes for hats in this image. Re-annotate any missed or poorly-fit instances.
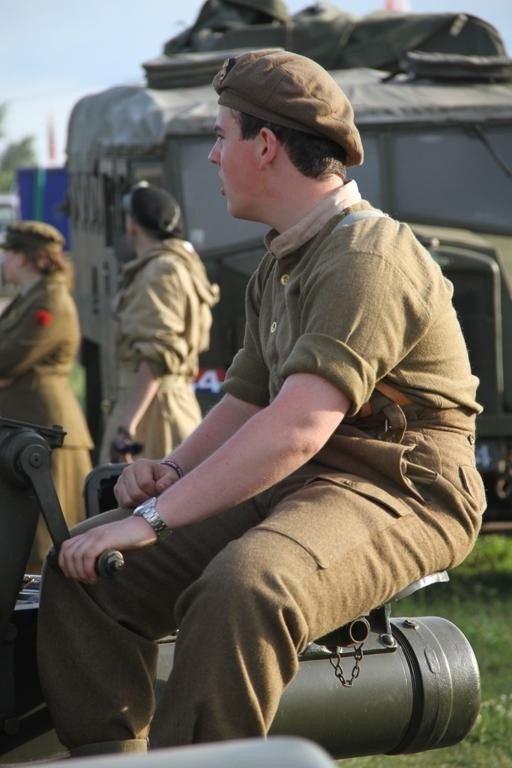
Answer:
[1,218,69,257]
[209,43,365,170]
[121,180,185,240]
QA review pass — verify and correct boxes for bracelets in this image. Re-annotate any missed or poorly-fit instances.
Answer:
[162,461,183,478]
[115,425,136,440]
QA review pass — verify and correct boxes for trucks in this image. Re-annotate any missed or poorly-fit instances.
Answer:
[66,68,511,534]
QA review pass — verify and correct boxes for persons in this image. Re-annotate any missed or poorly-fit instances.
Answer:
[97,181,220,469]
[1,219,95,578]
[36,49,491,754]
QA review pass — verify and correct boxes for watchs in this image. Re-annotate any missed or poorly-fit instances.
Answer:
[133,495,172,542]
